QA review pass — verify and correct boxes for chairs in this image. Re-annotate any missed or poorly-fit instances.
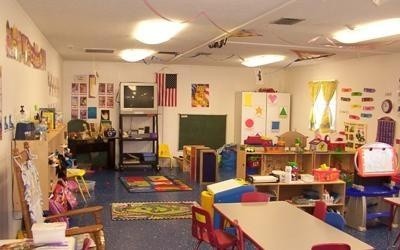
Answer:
[8,149,107,250]
[190,192,374,250]
[157,143,174,169]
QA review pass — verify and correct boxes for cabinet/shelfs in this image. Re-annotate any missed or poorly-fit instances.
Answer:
[11,124,66,213]
[232,87,397,231]
[119,114,160,172]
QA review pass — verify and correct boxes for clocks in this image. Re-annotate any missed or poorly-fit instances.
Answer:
[381,99,393,113]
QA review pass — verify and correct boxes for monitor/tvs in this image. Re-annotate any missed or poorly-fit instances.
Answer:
[119,82,158,114]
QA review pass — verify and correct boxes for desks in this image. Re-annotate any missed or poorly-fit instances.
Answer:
[384,196,400,250]
[67,138,119,172]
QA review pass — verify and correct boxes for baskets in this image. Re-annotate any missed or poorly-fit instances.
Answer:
[314,169,340,182]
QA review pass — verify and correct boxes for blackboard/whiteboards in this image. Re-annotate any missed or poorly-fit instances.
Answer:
[179,114,227,150]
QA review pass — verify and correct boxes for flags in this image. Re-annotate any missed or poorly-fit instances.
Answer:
[157,72,177,107]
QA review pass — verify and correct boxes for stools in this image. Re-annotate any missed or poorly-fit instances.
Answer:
[65,168,92,203]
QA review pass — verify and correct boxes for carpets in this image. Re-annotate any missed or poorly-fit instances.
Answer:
[109,200,202,220]
[118,173,195,194]
